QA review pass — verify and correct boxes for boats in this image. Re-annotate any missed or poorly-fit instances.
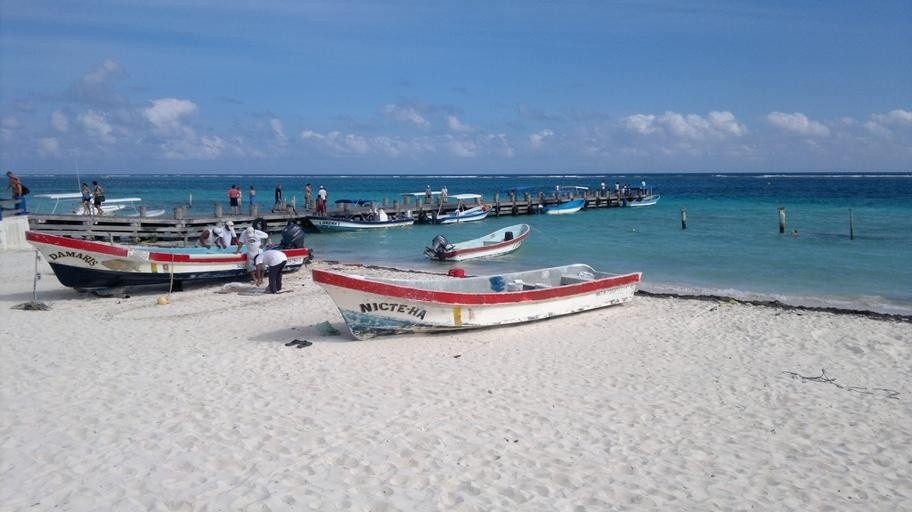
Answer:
[22,228,311,289]
[31,192,165,216]
[309,260,642,346]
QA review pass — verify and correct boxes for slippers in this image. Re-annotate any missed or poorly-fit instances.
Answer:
[285,339,313,349]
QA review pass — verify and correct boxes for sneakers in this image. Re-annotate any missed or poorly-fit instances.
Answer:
[83,210,105,215]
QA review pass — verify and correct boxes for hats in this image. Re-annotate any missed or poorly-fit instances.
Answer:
[319,185,323,189]
[213,226,224,238]
[226,221,235,233]
[247,227,255,240]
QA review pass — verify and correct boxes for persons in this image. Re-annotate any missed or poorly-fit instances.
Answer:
[237,186,242,214]
[248,184,256,205]
[440,184,448,206]
[226,185,240,216]
[272,184,282,213]
[426,184,433,204]
[319,186,327,211]
[600,178,646,198]
[5,171,22,196]
[80,180,105,216]
[315,194,324,217]
[304,184,313,211]
[792,230,799,237]
[194,219,288,295]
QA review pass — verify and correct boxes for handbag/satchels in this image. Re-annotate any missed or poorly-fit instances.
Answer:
[98,196,106,202]
[17,185,30,196]
